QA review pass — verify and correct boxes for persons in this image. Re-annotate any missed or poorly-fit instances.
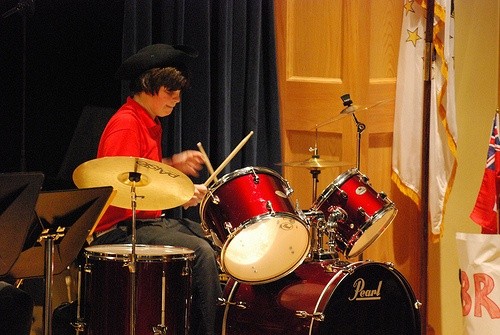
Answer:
[86,44,224,335]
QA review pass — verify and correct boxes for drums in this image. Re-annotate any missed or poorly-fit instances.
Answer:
[222,258,422,335]
[77,245,196,335]
[312,169,398,258]
[199,167,311,284]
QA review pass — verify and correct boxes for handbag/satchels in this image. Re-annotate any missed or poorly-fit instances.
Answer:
[456,208,500,335]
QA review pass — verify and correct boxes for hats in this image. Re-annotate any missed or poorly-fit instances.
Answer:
[114,44,193,81]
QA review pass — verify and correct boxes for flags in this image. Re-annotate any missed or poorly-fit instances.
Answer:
[469,110,500,234]
[391,0,458,244]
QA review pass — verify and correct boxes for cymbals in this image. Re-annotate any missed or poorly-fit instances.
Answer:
[273,157,357,168]
[73,157,195,210]
[311,101,382,131]
